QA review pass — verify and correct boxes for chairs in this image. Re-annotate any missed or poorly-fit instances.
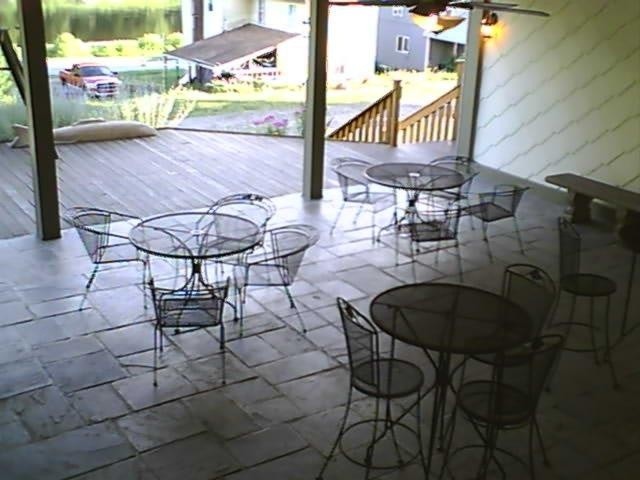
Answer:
[173,193,277,304]
[436,264,564,480]
[61,207,156,314]
[134,277,231,387]
[416,156,480,233]
[545,217,621,393]
[394,203,462,282]
[315,297,430,480]
[233,224,320,338]
[329,157,396,245]
[458,184,530,263]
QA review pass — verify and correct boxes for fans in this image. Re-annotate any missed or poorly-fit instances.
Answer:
[331,0,550,25]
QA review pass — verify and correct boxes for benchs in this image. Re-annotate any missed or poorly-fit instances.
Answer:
[545,174,640,248]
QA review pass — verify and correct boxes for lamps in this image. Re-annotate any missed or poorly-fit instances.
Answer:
[477,10,500,39]
[411,5,468,39]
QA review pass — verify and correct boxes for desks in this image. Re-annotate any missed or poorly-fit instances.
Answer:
[128,212,263,334]
[363,163,464,252]
[366,283,533,480]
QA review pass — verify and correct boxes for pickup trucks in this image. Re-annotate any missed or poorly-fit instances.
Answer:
[56,62,122,101]
